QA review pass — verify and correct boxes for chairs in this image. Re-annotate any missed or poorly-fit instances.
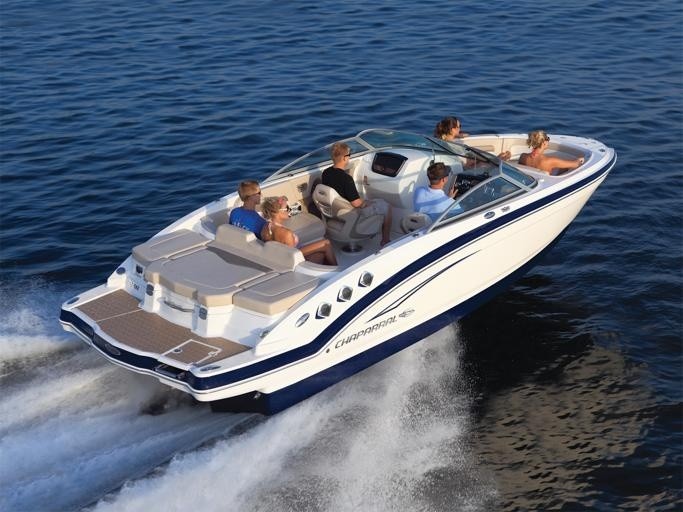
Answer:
[213,184,432,269]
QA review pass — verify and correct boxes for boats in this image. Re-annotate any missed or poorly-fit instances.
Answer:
[59,128,617,416]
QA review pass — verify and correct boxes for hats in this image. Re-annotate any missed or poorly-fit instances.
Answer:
[427,162,451,179]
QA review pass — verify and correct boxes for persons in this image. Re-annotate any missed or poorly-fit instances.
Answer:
[411,161,463,224]
[258,196,338,266]
[321,142,392,246]
[228,180,264,240]
[432,115,511,171]
[517,129,583,176]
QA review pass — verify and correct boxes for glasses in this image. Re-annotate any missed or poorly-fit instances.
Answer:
[248,191,262,196]
[344,154,350,157]
[456,124,461,128]
[545,137,550,141]
[279,205,289,212]
